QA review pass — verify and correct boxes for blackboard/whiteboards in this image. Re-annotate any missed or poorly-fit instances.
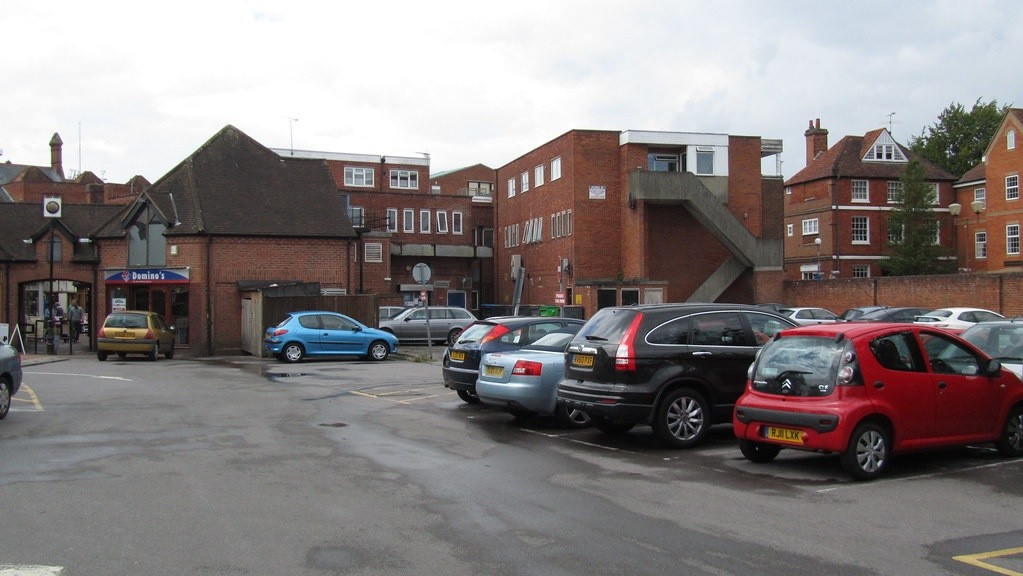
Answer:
[0,323,9,345]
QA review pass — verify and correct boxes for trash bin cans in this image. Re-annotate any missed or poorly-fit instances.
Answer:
[538,305,560,318]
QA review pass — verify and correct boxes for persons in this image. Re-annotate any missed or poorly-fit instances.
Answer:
[55,302,65,335]
[43,305,52,338]
[68,298,83,343]
[31,302,39,333]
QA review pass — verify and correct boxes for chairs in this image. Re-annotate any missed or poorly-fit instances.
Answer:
[1001,333,1023,357]
[535,328,546,339]
[721,320,745,345]
[876,339,908,371]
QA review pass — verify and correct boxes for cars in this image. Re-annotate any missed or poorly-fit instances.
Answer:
[442,315,590,405]
[474,325,600,429]
[854,307,934,323]
[762,307,839,340]
[265,310,400,365]
[0,342,23,420]
[904,326,1003,364]
[379,305,406,321]
[377,306,487,348]
[746,303,795,322]
[931,319,1023,384]
[841,306,888,322]
[96,310,176,362]
[733,323,1023,485]
[911,308,1005,329]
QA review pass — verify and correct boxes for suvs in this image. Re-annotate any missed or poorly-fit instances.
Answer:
[557,302,812,450]
